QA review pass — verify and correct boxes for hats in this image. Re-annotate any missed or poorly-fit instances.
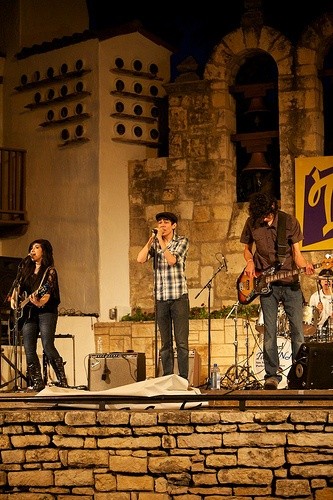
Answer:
[155,212,178,224]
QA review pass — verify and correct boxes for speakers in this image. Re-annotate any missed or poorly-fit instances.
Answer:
[158,356,199,387]
[88,353,146,391]
[287,342,333,389]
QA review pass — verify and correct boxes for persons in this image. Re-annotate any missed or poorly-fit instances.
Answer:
[137,211,189,383]
[10,239,70,391]
[240,193,333,390]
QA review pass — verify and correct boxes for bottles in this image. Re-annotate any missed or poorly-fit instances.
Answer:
[210,363,220,390]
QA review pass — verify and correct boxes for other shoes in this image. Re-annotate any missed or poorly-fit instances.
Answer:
[264,376,280,389]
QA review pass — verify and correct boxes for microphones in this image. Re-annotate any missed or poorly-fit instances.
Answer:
[223,255,228,271]
[102,366,107,380]
[152,228,158,233]
[17,252,33,267]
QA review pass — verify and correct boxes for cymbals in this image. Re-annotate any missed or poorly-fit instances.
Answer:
[308,274,333,280]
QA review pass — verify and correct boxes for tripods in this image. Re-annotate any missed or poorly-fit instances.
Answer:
[219,302,263,389]
[194,263,227,390]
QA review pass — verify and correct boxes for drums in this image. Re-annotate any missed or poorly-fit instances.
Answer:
[254,304,286,335]
[285,303,321,335]
[305,327,333,343]
[251,336,293,389]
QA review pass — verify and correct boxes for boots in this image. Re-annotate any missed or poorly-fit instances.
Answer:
[28,361,45,392]
[50,356,69,388]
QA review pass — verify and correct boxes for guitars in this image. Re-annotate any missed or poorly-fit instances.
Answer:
[9,283,50,332]
[236,257,333,305]
